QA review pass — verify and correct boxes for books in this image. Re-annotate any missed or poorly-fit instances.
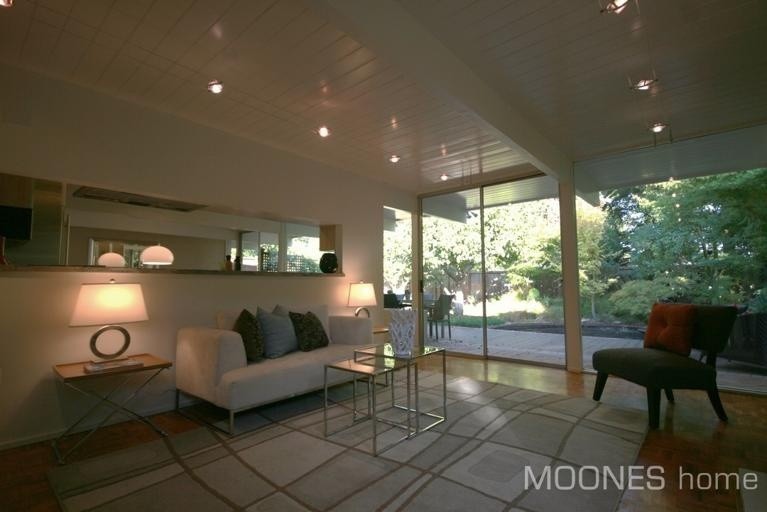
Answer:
[83,356,144,375]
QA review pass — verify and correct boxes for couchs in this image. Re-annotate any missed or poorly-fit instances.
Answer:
[170,311,374,435]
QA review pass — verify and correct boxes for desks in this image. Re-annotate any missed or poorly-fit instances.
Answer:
[373,329,390,334]
[53,353,173,464]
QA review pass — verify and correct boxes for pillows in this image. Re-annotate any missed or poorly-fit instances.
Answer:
[214,307,239,329]
[643,303,697,357]
[257,303,297,358]
[235,309,264,360]
[289,310,330,351]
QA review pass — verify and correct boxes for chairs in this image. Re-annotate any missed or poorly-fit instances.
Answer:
[593,301,743,431]
[384,294,454,341]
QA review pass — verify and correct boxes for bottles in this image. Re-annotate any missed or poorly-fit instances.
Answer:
[225,255,241,271]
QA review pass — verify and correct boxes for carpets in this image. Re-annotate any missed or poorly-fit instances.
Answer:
[44,367,649,512]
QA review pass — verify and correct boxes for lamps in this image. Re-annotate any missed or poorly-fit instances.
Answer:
[347,282,378,318]
[70,279,150,363]
[98,252,126,268]
[137,245,173,265]
